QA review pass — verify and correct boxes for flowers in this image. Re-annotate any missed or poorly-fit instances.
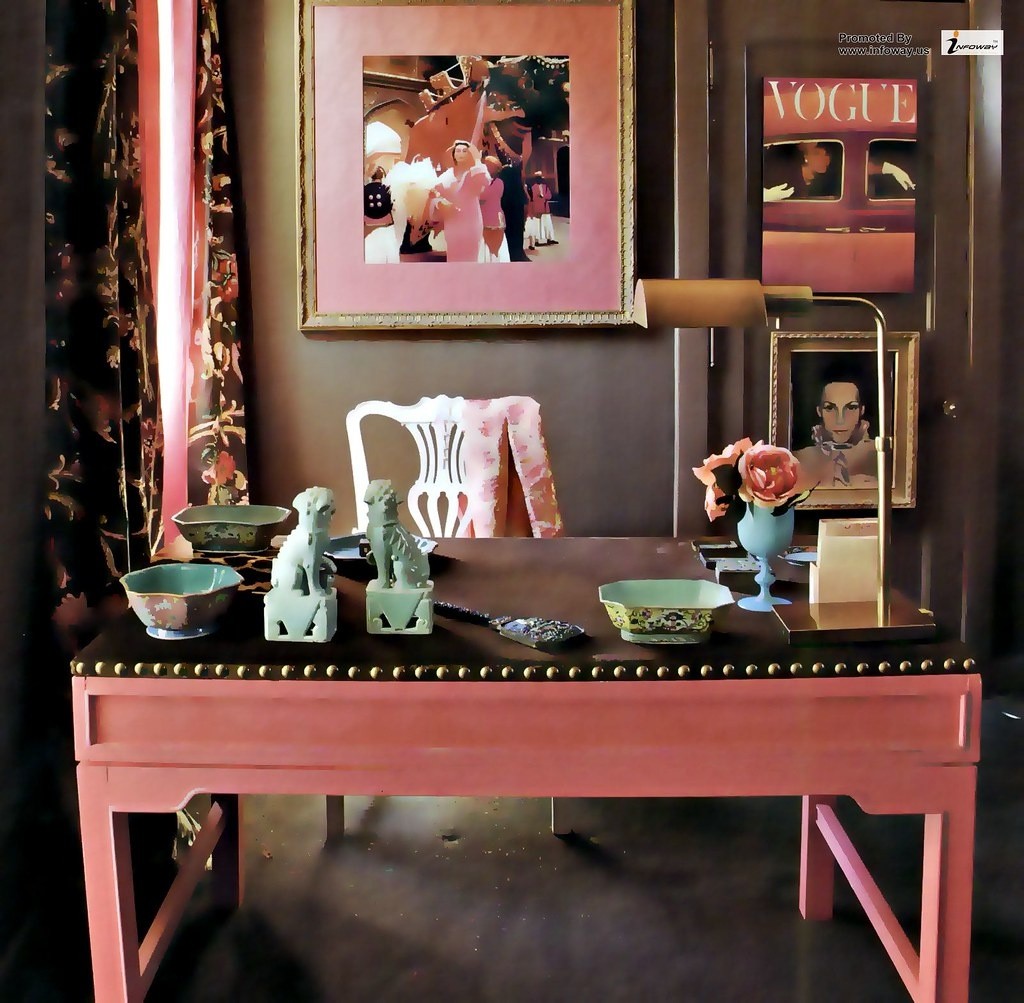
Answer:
[691,435,821,521]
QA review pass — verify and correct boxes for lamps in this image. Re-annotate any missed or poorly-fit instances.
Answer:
[625,276,941,651]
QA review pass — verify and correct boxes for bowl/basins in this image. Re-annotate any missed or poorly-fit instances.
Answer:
[118,563,245,640]
[598,579,736,645]
[170,504,293,553]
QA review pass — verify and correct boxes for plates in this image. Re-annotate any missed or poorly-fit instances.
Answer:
[322,530,440,568]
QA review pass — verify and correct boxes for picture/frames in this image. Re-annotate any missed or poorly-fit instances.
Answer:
[294,0,638,331]
[743,39,933,308]
[767,330,922,509]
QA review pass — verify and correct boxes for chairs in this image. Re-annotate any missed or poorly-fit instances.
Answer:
[344,392,570,539]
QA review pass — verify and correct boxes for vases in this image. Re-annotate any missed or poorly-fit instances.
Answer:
[736,504,794,613]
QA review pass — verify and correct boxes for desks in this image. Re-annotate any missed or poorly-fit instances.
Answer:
[69,534,984,1003]
